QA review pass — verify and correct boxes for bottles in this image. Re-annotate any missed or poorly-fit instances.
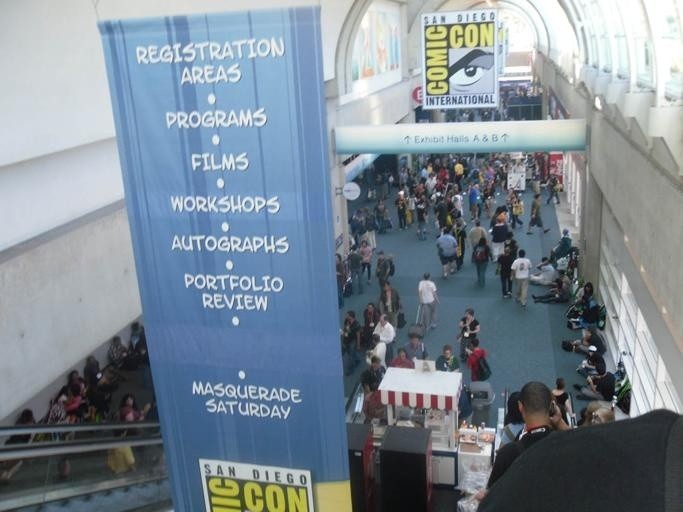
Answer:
[460,419,486,442]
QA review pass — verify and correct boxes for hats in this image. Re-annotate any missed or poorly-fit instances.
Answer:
[588,345,598,352]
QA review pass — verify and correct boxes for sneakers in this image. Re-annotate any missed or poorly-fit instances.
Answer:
[502,290,537,308]
[441,266,462,279]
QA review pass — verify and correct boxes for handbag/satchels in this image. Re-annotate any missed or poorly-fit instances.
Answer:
[511,198,525,217]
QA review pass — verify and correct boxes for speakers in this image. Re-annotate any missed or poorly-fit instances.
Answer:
[380,426,432,512]
[346,423,375,512]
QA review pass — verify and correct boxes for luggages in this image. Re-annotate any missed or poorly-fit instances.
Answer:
[407,303,426,339]
[341,262,353,298]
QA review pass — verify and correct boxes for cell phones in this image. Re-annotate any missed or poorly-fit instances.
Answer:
[549,403,554,419]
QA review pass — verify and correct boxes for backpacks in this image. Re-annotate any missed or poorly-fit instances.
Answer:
[383,258,396,277]
[472,353,492,382]
[472,245,489,263]
[491,224,507,243]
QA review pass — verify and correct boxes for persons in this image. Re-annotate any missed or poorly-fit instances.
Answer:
[445,88,528,122]
[334,155,628,427]
[1,321,164,483]
[475,382,573,501]
